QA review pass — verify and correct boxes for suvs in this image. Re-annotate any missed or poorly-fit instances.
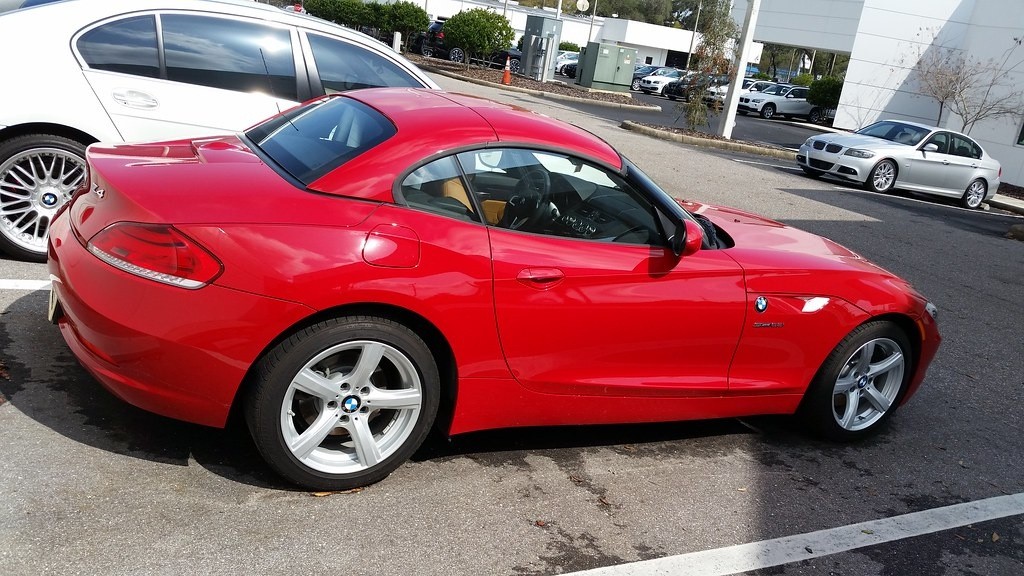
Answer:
[738,82,826,124]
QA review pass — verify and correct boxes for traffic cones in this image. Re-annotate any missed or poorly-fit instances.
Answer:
[500,57,511,85]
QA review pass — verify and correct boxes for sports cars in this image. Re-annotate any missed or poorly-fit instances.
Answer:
[46,88,942,493]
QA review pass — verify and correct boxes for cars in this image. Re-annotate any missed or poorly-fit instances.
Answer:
[665,74,735,103]
[639,70,699,97]
[424,22,523,73]
[0,0,622,262]
[556,51,581,79]
[701,78,785,116]
[630,66,680,91]
[402,20,436,59]
[795,119,1002,210]
